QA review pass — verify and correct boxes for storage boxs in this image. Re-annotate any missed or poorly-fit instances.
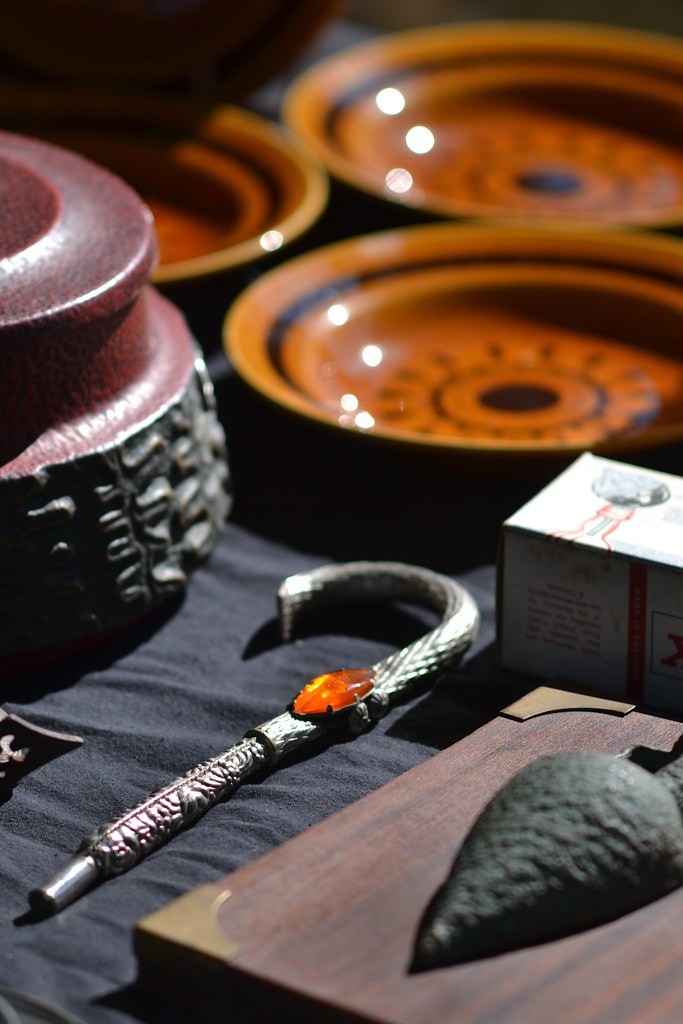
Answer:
[494,449,682,716]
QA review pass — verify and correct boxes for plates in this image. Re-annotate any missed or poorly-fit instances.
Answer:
[219,211,683,466]
[278,11,683,240]
[0,71,330,298]
